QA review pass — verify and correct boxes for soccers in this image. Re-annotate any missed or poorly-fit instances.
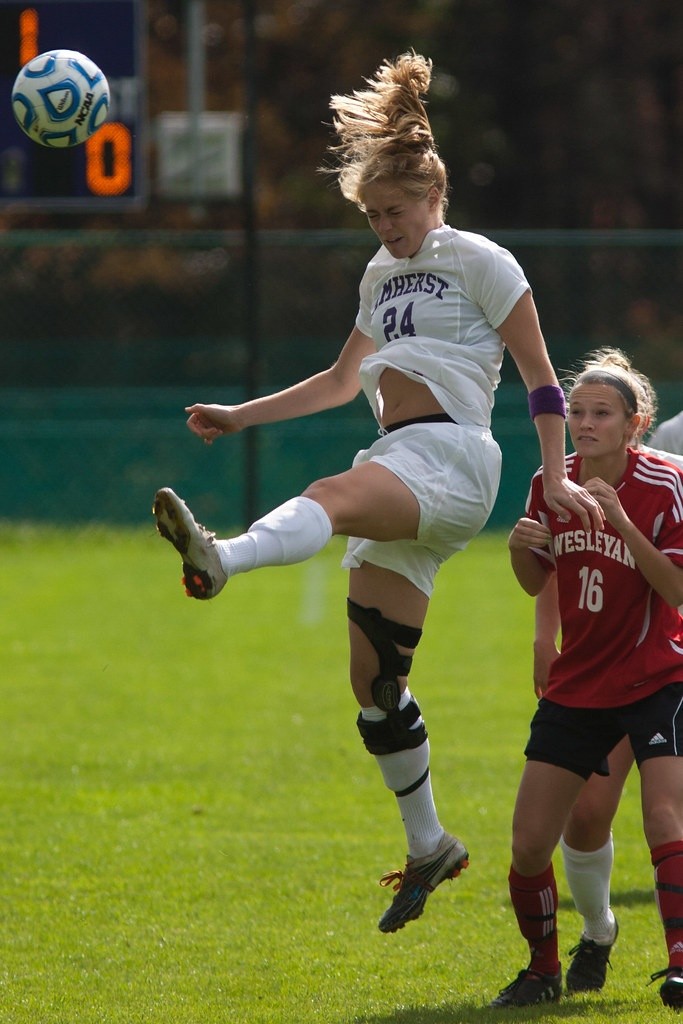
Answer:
[12,48,113,150]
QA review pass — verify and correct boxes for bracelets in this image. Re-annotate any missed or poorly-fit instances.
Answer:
[528,385,566,421]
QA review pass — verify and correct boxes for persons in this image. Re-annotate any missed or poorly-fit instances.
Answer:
[150,47,602,938]
[487,342,683,1012]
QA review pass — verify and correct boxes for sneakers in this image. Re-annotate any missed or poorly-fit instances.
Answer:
[490,961,563,1009]
[378,831,469,933]
[646,966,683,1008]
[153,488,228,600]
[566,905,619,994]
